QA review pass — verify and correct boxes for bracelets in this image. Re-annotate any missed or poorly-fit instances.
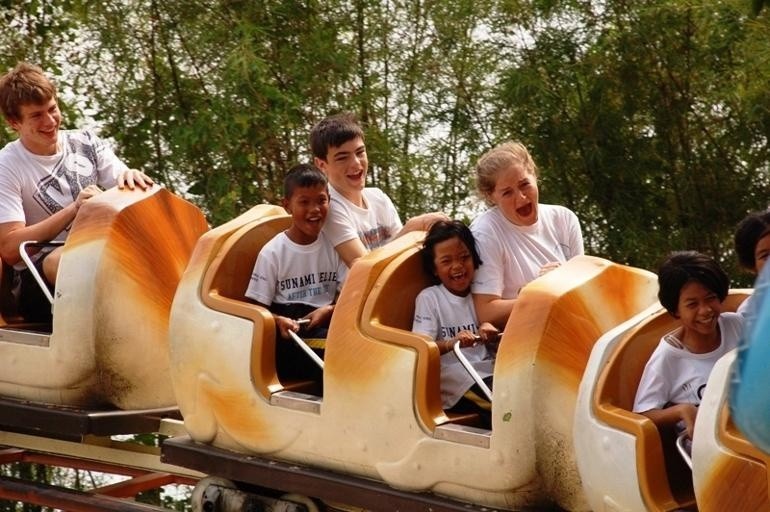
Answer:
[443,338,452,355]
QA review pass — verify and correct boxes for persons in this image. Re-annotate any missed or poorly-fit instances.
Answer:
[407,219,501,426]
[244,162,351,399]
[464,139,587,334]
[734,207,770,325]
[308,111,454,294]
[0,61,157,321]
[631,248,745,455]
